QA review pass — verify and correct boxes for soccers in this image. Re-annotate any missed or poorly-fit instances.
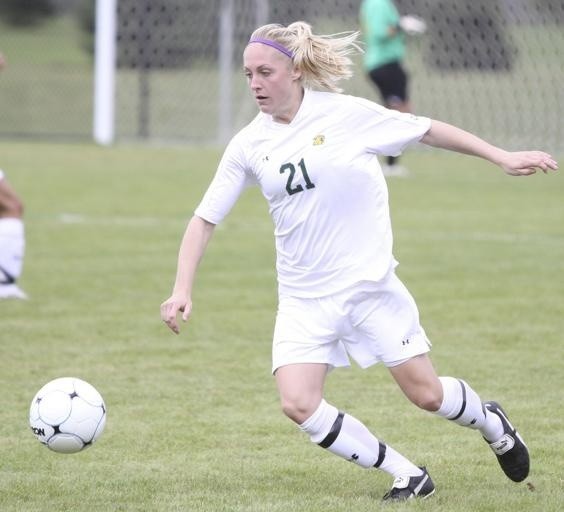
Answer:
[28,375,106,454]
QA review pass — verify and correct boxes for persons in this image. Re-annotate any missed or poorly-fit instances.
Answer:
[0,167,29,299]
[359,0,428,176]
[160,22,558,503]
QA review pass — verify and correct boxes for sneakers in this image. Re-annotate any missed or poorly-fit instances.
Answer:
[481,400,530,483]
[381,465,436,502]
[0,284,27,300]
